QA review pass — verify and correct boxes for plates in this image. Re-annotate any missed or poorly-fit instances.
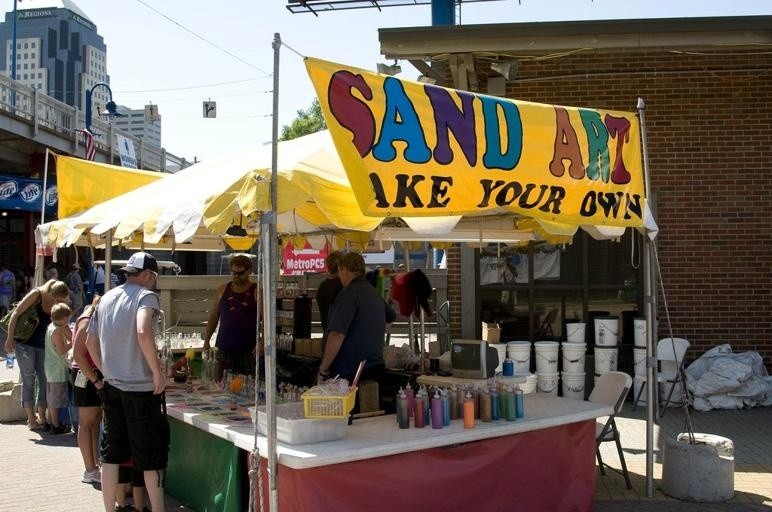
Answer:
[276,297,312,353]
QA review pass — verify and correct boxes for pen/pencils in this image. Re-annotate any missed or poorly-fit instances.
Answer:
[92,259,184,277]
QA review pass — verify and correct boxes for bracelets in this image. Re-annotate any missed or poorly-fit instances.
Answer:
[93,377,99,384]
[319,366,327,376]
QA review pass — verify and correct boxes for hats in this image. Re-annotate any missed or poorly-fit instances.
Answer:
[614,276,637,305]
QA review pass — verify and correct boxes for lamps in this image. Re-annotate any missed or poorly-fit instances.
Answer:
[589,372,633,489]
[632,338,691,418]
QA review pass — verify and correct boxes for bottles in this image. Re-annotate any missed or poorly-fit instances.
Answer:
[72,263,82,269]
[118,251,159,275]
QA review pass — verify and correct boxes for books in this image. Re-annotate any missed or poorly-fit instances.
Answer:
[295,339,323,357]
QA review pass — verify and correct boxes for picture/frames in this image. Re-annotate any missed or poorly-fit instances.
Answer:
[416,374,526,404]
[236,393,615,512]
[166,385,262,512]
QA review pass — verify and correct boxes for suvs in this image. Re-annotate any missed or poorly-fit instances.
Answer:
[488,315,686,408]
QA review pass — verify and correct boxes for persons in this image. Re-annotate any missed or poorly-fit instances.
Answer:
[315,254,396,407]
[203,254,264,380]
[2,269,35,315]
[4,279,69,430]
[43,303,75,433]
[48,260,119,324]
[72,295,101,483]
[85,251,168,511]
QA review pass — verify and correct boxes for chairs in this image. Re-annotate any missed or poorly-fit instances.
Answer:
[114,500,151,512]
[42,421,72,435]
[27,415,47,430]
[81,467,101,483]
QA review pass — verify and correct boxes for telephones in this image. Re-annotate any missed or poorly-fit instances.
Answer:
[282,242,329,275]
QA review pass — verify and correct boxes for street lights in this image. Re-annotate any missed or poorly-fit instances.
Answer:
[73,369,89,389]
[0,288,42,344]
[70,290,83,309]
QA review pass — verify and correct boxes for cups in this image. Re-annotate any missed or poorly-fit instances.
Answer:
[148,268,158,280]
[229,268,250,276]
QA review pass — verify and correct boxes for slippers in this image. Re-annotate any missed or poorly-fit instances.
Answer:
[2,270,14,289]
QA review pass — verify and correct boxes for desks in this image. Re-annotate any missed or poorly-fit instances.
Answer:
[9,0,24,113]
[81,78,125,161]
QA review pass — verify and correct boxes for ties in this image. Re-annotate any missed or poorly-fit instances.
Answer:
[450,339,499,379]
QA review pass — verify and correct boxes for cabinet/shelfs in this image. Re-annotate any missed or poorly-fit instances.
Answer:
[277,281,299,296]
[7,351,14,370]
[396,381,524,431]
[414,333,419,358]
[163,331,308,402]
[503,359,514,375]
[385,333,391,347]
